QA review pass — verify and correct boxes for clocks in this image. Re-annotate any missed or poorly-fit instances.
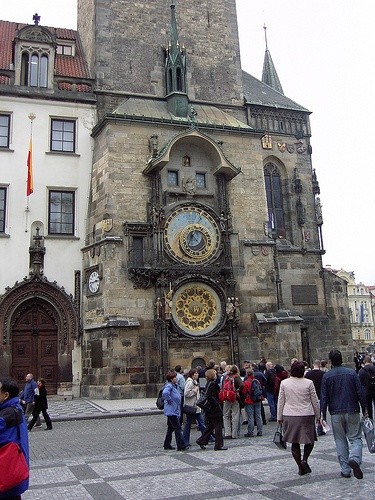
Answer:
[87,269,101,296]
[160,201,225,268]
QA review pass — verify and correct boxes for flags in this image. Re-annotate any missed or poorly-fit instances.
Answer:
[26,136,33,196]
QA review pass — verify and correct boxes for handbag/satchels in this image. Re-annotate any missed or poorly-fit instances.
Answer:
[0,442,29,491]
[196,381,212,411]
[183,405,197,415]
[360,416,375,454]
[273,423,286,450]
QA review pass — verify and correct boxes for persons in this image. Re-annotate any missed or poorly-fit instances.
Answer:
[276,361,320,477]
[0,377,29,500]
[320,350,367,479]
[27,378,52,432]
[19,373,42,428]
[291,358,330,437]
[162,355,288,451]
[354,352,375,420]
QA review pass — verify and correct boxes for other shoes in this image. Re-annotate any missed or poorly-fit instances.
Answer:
[268,417,277,422]
[263,421,267,425]
[196,439,206,449]
[164,445,175,450]
[208,438,215,443]
[257,432,262,436]
[299,468,305,475]
[301,462,312,473]
[27,428,31,432]
[341,472,349,477]
[348,460,363,479]
[177,446,189,451]
[33,424,42,429]
[244,433,254,437]
[214,446,228,450]
[242,421,248,425]
[223,434,232,439]
[196,426,200,431]
[44,427,52,430]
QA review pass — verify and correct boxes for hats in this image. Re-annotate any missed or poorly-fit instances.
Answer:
[244,360,252,363]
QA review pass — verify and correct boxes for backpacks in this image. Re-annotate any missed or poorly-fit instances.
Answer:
[219,374,237,402]
[246,378,262,403]
[156,383,173,410]
